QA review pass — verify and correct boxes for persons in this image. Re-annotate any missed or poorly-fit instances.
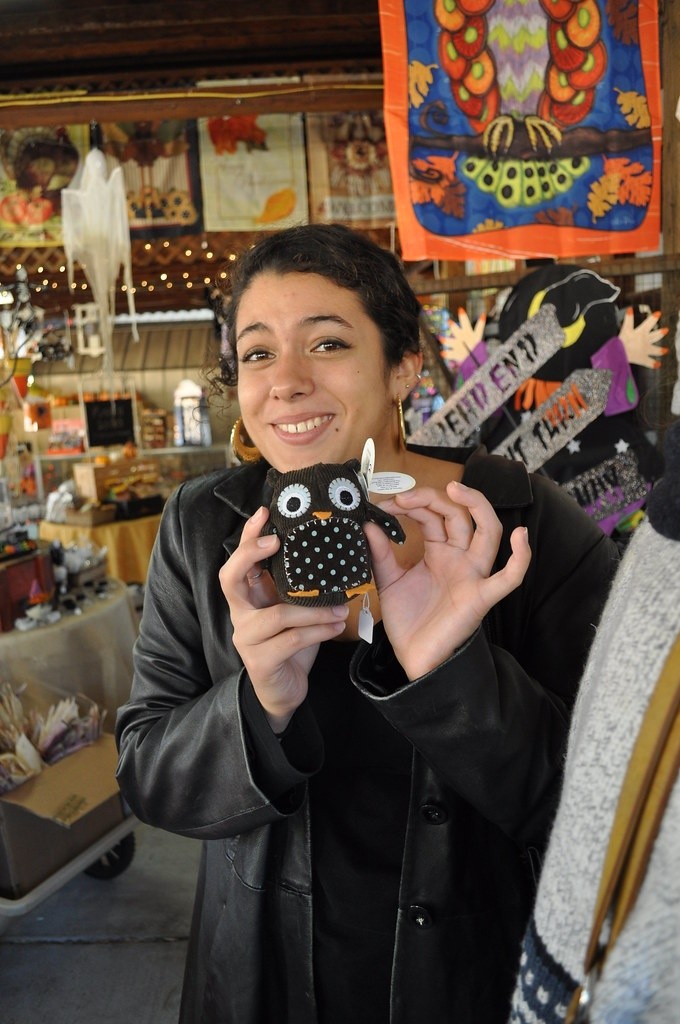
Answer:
[113,221,621,1021]
